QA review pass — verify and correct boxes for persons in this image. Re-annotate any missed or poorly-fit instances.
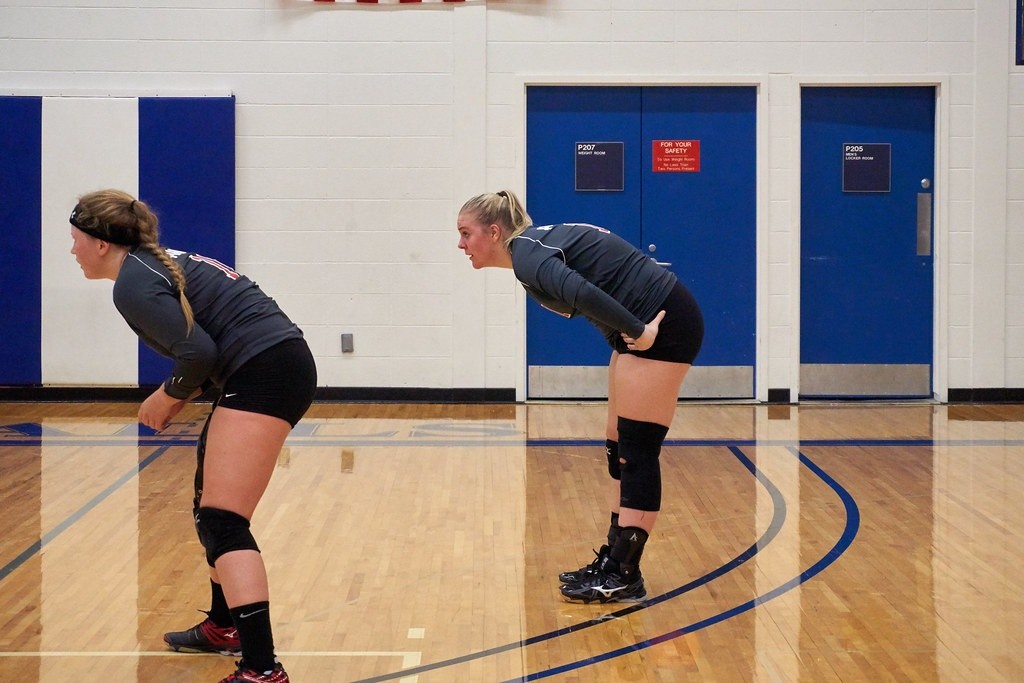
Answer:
[68,189,317,683]
[457,189,705,604]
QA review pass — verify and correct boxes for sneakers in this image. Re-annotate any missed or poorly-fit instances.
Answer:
[161,609,243,657]
[217,657,290,683]
[558,554,647,603]
[558,544,609,586]
